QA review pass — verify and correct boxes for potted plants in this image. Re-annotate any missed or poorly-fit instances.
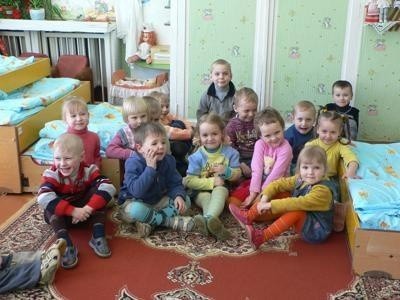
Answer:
[23,0,63,20]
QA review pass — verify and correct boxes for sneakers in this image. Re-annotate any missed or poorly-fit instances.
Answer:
[136,221,152,238]
[88,234,112,257]
[245,225,265,251]
[172,215,231,240]
[229,204,252,229]
[333,203,345,232]
[37,238,79,285]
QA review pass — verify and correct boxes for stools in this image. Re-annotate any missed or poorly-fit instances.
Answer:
[108,78,170,105]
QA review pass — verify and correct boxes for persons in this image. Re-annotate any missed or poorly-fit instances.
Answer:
[0,238,66,294]
[38,98,116,268]
[106,59,360,252]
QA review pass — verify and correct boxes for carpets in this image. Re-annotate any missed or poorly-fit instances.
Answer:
[0,197,400,300]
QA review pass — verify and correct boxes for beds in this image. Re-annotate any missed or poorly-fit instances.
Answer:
[338,141,400,279]
[0,55,128,196]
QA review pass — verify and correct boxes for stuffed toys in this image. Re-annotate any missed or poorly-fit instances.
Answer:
[127,23,156,64]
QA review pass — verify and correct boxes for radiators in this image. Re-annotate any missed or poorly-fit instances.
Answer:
[4,31,107,88]
